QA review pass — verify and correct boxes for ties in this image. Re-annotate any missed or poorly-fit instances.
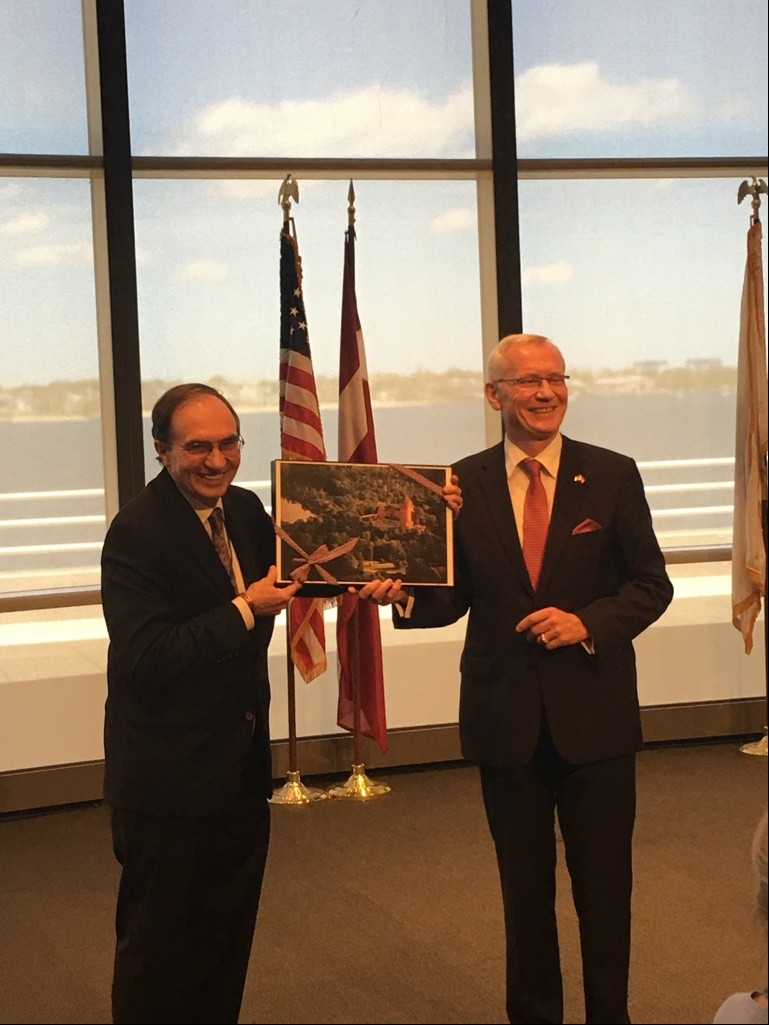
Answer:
[518,459,551,593]
[208,507,233,577]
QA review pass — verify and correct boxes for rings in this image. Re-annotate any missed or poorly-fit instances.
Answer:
[542,633,547,643]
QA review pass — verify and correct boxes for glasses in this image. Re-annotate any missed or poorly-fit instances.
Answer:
[166,434,245,454]
[493,373,571,388]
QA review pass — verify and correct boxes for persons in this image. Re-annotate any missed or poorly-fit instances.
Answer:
[349,335,674,1024]
[99,385,300,1025]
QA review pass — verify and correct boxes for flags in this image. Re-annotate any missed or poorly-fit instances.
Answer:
[280,221,328,686]
[336,224,389,754]
[733,219,768,654]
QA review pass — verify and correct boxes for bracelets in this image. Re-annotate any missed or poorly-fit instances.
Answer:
[238,592,255,616]
